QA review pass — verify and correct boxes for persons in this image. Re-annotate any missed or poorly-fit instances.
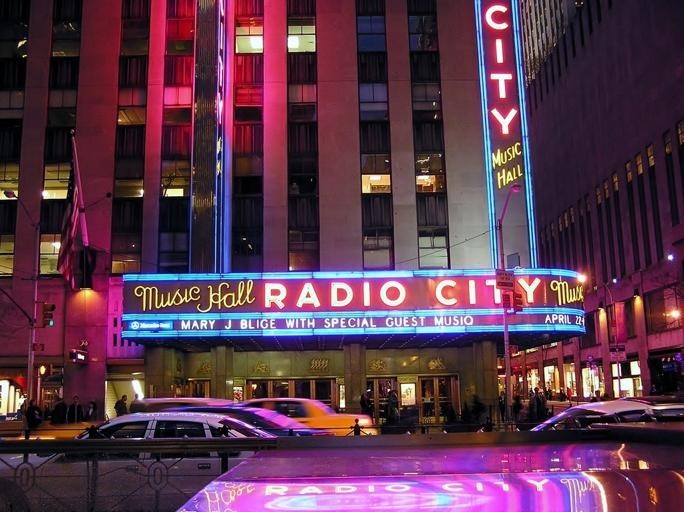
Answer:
[114,395,130,412]
[497,390,506,422]
[590,390,606,403]
[21,394,100,428]
[544,386,573,402]
[359,389,372,414]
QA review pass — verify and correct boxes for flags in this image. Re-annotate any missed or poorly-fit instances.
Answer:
[55,138,90,289]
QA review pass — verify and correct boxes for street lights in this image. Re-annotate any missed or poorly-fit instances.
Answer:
[3,187,42,405]
[576,272,621,397]
[498,182,523,432]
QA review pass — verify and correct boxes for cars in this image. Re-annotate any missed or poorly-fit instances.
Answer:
[530,397,684,432]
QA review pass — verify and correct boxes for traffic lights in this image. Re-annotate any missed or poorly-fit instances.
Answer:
[513,292,523,312]
[42,302,56,328]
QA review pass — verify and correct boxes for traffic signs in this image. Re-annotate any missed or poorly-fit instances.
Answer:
[496,269,516,291]
